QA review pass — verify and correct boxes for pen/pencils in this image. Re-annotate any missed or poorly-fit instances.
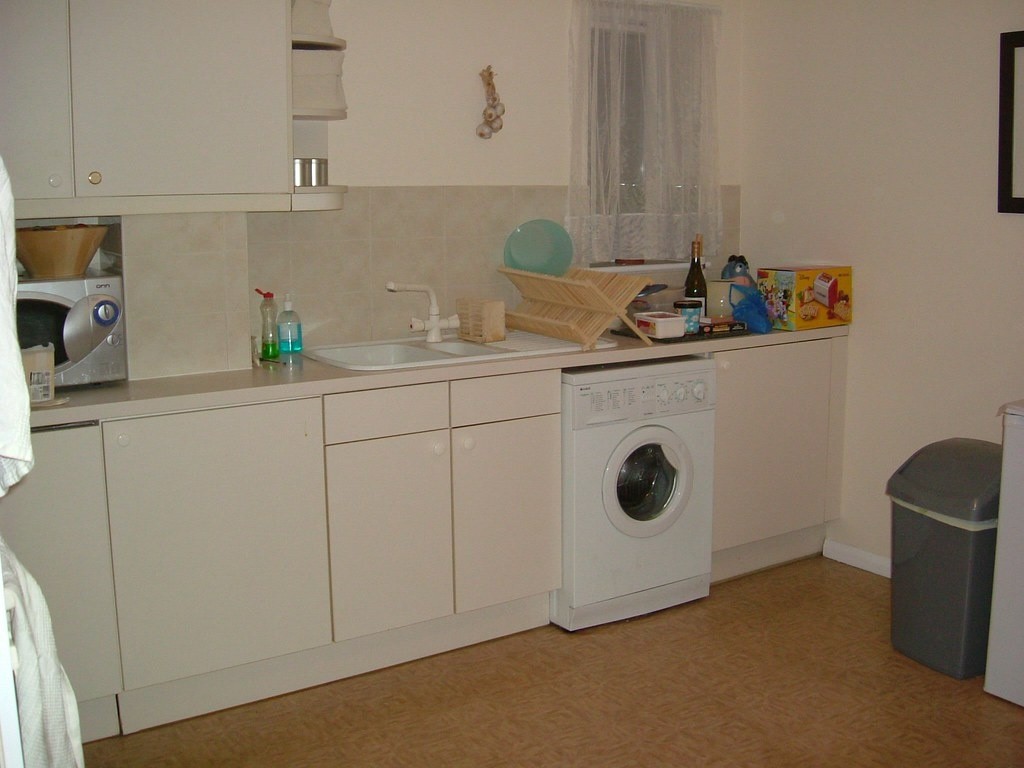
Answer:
[259,357,286,365]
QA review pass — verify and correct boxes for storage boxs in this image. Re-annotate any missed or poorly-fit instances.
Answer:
[634,311,688,339]
[757,263,853,331]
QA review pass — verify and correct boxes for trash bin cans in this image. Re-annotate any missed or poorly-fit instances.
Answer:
[885,437,1002,680]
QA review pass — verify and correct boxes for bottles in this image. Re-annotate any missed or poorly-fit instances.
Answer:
[684,241,708,320]
[255,288,279,359]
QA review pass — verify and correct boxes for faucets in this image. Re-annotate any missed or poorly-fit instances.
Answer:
[386,282,462,348]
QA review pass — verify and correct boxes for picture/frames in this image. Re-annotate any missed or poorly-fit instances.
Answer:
[998,30,1024,213]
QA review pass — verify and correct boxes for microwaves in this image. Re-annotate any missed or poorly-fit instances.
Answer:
[13,267,128,387]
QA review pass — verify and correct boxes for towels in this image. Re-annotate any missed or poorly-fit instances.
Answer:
[0,158,33,498]
[1,538,86,768]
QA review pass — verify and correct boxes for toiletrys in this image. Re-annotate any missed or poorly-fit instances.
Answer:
[279,292,301,352]
[254,288,277,360]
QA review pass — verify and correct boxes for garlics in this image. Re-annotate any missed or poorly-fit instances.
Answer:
[476,103,505,139]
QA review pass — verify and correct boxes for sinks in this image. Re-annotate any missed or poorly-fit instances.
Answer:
[428,340,499,357]
[304,340,447,371]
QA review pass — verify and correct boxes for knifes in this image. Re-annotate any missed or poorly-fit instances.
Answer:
[614,258,680,265]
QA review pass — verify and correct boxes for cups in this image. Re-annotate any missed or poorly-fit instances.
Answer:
[706,279,741,317]
[295,158,329,187]
[673,300,702,334]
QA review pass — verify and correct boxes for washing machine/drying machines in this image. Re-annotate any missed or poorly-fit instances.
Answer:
[550,360,716,630]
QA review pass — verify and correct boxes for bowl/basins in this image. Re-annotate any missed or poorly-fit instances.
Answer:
[12,223,108,280]
[502,218,574,277]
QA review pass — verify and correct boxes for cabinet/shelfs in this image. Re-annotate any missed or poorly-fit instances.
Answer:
[696,336,848,586]
[1,422,124,744]
[0,0,349,219]
[100,395,334,736]
[323,368,563,678]
